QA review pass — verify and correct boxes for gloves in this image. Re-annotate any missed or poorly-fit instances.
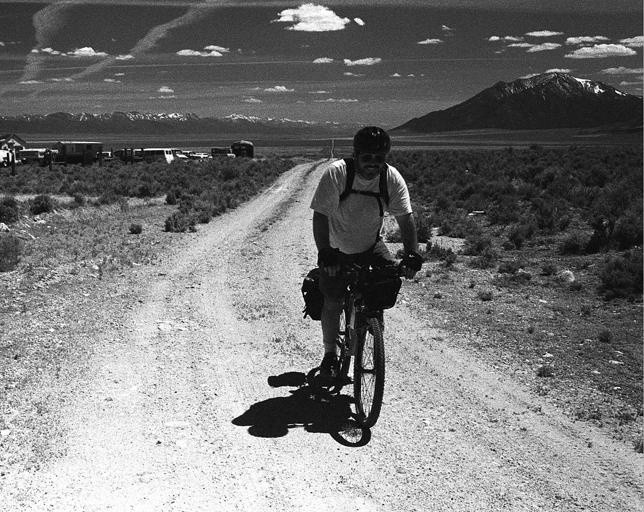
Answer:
[318,248,342,267]
[401,250,424,272]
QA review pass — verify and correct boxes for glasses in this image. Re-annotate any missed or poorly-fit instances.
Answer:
[360,153,385,163]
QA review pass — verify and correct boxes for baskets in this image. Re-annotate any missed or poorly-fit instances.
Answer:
[357,276,402,309]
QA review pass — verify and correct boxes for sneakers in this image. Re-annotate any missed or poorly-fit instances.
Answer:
[320,357,340,379]
[367,309,384,335]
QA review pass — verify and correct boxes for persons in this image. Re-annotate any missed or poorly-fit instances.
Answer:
[310,126,425,378]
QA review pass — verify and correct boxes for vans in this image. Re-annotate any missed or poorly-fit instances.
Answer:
[1,140,253,165]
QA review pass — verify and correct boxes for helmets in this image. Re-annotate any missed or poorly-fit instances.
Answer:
[353,126,391,155]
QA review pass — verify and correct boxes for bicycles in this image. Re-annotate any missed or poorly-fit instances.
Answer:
[330,260,412,429]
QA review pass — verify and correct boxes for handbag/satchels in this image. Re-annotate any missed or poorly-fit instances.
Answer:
[302,268,323,320]
